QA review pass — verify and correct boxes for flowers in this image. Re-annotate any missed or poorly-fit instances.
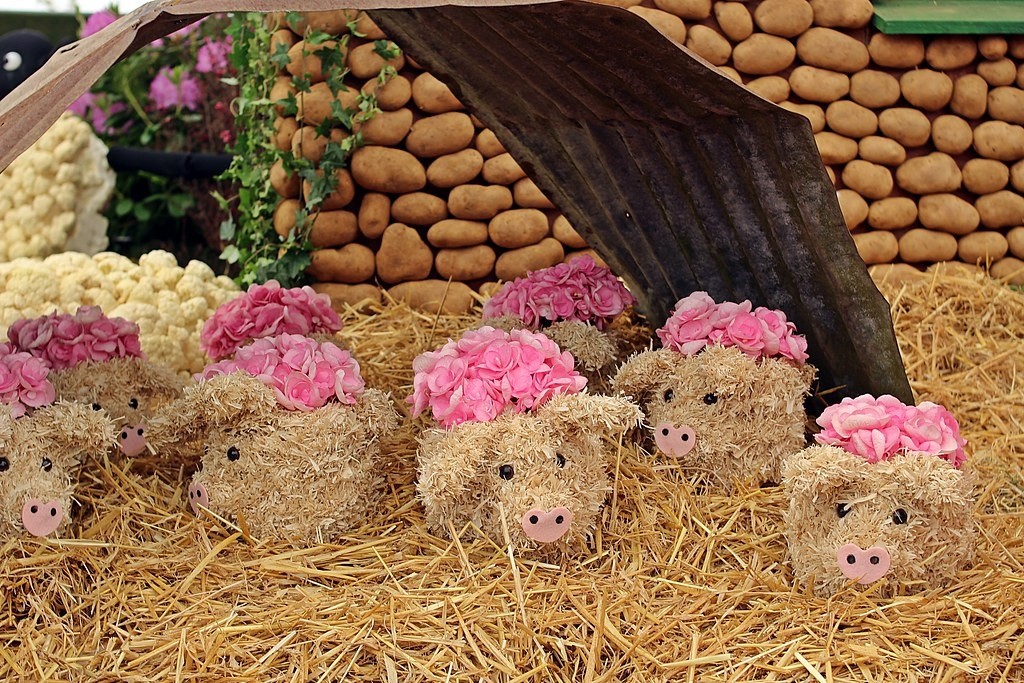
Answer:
[813,394,969,469]
[198,280,343,363]
[191,334,367,413]
[0,340,58,421]
[57,10,237,146]
[656,290,811,368]
[483,256,635,335]
[6,304,149,373]
[406,326,591,430]
